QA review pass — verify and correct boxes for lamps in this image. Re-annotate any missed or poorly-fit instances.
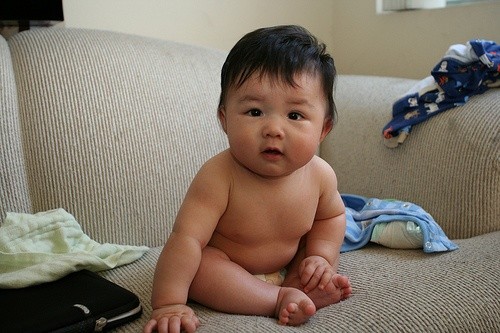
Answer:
[0,0,66,34]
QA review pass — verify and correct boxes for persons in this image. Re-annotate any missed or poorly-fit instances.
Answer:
[143,24,351,333]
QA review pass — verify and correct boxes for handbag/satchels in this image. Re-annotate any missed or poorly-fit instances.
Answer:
[0,267,142,333]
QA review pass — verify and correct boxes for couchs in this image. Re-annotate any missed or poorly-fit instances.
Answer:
[0,23,500,333]
